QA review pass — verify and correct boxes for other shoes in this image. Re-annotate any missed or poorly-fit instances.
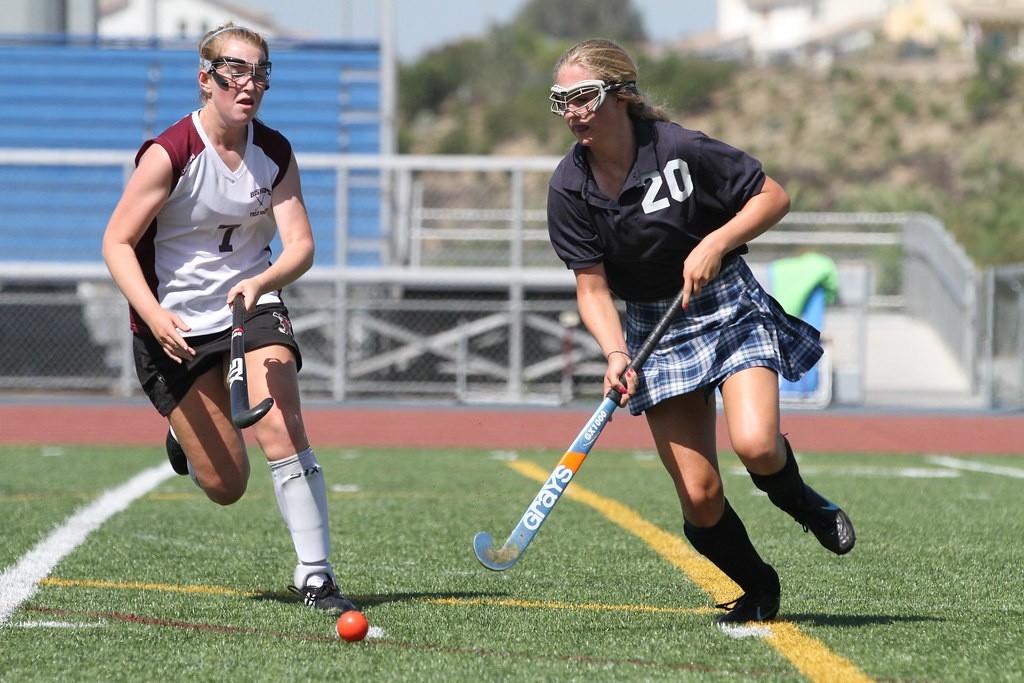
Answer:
[166,423,190,475]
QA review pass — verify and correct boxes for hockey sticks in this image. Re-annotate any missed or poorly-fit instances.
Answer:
[471,280,688,571]
[222,291,274,431]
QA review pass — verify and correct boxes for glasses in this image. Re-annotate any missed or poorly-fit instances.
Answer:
[549,80,637,118]
[199,56,272,91]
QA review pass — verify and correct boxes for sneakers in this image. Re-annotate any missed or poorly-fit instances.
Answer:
[287,571,356,614]
[716,590,781,623]
[786,483,855,555]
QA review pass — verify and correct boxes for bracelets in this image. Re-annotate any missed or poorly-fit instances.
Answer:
[605,349,632,360]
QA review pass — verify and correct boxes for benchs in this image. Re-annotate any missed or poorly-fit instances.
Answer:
[0,33,384,263]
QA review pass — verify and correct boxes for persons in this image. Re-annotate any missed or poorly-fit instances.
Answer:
[547,39,856,624]
[102,22,358,617]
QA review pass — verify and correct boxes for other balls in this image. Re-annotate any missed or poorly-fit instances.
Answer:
[337,608,370,641]
